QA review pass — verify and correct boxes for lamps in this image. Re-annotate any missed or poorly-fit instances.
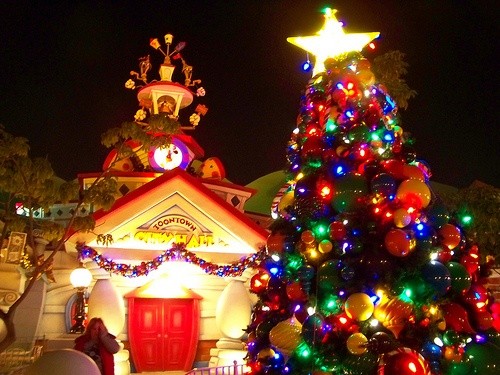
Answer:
[70,259,91,333]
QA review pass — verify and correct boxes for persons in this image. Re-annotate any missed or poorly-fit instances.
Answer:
[72,317,119,375]
